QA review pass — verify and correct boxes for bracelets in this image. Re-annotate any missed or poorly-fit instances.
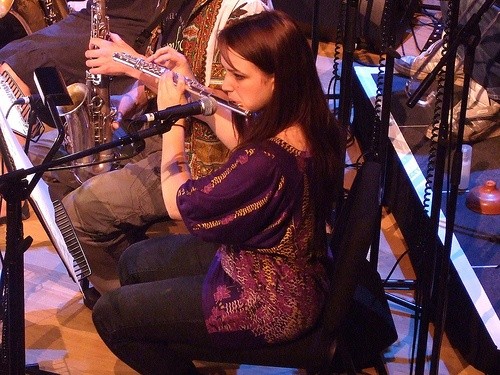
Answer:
[173,123,186,129]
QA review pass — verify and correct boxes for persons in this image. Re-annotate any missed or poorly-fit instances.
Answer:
[0,0,343,375]
[394,0,499,145]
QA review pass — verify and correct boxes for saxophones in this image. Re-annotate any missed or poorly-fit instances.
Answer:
[56,0,122,182]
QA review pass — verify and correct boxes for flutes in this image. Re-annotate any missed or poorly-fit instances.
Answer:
[111,52,255,118]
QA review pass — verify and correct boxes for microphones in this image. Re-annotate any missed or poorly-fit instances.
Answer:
[135,97,217,122]
[14,94,42,104]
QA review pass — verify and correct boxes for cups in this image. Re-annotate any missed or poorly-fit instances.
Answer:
[458,144,473,193]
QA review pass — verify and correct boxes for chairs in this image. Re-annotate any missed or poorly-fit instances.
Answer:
[167,162,382,375]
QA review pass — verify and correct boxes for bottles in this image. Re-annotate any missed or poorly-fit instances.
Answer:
[405,4,500,108]
